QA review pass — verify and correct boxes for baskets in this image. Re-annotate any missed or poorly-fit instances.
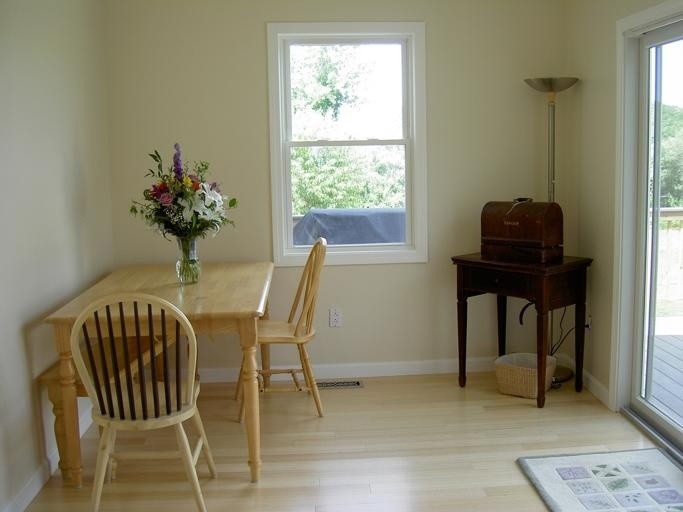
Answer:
[494,352,556,399]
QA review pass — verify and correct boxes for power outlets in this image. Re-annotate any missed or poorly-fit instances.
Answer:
[329,307,343,327]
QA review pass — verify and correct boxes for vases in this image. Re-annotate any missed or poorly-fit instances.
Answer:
[176,231,202,284]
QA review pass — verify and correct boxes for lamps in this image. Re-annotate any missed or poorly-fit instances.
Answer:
[524,75,579,384]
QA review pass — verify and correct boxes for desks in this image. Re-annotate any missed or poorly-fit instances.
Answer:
[43,259,276,488]
[449,251,596,410]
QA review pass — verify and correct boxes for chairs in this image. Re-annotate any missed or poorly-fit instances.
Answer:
[65,292,220,512]
[234,237,332,422]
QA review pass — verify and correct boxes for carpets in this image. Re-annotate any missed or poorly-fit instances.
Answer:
[516,446,682,512]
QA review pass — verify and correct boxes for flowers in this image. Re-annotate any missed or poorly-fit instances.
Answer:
[130,139,239,270]
